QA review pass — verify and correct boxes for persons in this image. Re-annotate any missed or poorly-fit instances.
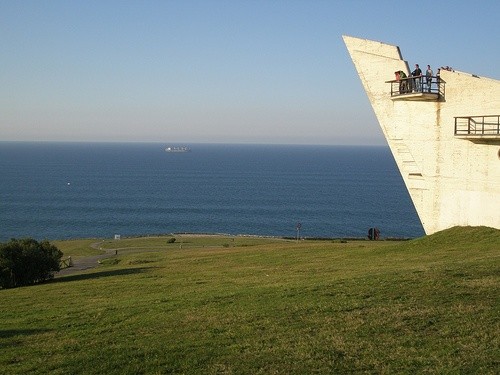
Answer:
[411,63,422,92]
[394,70,409,93]
[425,65,433,92]
[436,68,440,99]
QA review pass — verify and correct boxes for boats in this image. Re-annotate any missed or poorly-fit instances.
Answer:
[165,147,192,152]
